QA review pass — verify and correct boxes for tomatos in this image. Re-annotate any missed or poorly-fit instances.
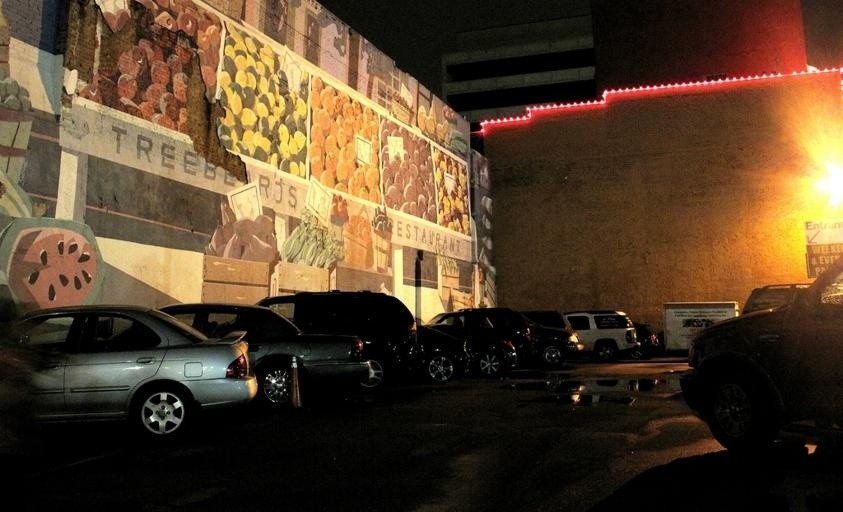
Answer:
[382,119,437,224]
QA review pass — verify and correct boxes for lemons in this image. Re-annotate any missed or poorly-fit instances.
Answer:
[221,19,307,179]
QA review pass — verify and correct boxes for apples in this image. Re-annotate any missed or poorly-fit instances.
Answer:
[116,0,221,132]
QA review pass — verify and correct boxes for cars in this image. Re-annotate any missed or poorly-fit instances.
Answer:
[679,253,843,451]
